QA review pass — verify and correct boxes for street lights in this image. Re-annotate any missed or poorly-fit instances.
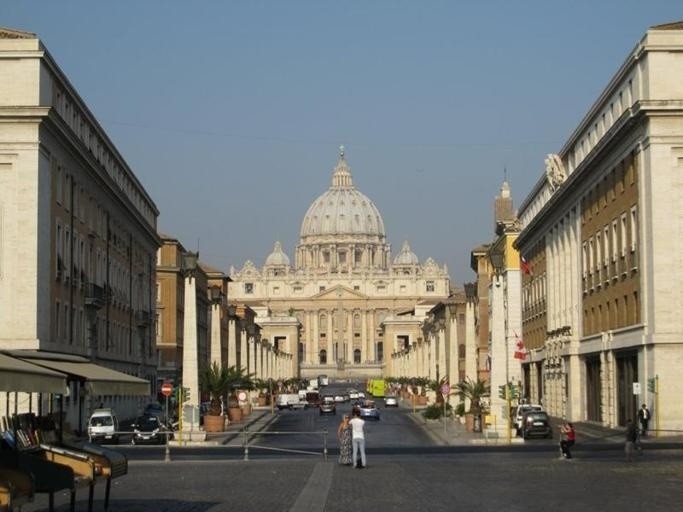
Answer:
[390,246,512,440]
[171,243,296,446]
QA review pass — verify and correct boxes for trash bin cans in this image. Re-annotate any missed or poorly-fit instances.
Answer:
[474,414,482,432]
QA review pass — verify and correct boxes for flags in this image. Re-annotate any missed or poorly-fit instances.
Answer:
[512,329,528,362]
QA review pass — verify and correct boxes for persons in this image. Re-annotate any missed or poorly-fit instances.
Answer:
[348,406,365,469]
[337,414,352,465]
[559,422,575,458]
[624,417,636,464]
[636,403,651,437]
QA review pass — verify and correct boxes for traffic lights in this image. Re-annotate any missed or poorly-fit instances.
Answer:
[498,385,504,398]
[647,378,655,393]
[512,384,518,399]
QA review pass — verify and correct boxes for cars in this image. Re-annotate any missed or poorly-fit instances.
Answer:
[510,403,552,439]
[85,401,209,444]
[274,374,398,421]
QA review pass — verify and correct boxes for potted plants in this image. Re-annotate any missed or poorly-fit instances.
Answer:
[454,402,466,424]
[269,377,308,401]
[225,364,268,424]
[449,376,491,433]
[384,375,454,424]
[198,360,237,433]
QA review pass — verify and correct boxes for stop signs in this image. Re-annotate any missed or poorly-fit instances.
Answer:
[162,383,172,396]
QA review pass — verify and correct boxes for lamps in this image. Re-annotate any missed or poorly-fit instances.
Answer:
[546,326,572,338]
